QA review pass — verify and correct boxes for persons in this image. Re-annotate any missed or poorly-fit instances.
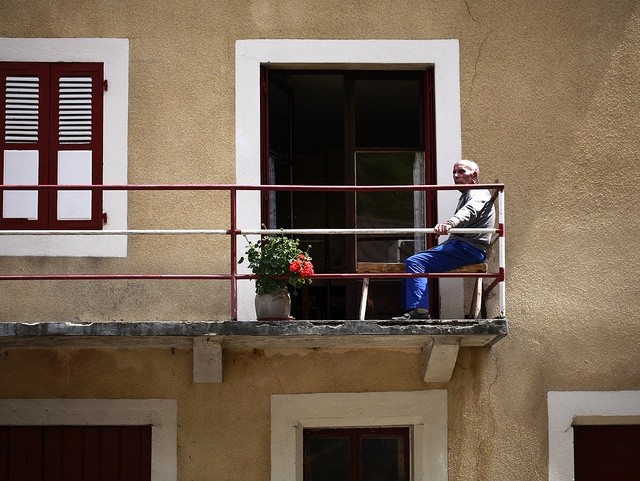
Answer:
[390,159,496,322]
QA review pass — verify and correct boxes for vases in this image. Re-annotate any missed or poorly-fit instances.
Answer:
[254,287,293,321]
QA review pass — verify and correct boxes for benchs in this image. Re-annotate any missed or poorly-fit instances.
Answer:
[357,262,487,321]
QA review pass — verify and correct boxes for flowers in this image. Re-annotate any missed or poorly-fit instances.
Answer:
[238,223,314,294]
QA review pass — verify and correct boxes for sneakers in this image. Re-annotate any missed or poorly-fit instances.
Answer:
[391,308,431,320]
